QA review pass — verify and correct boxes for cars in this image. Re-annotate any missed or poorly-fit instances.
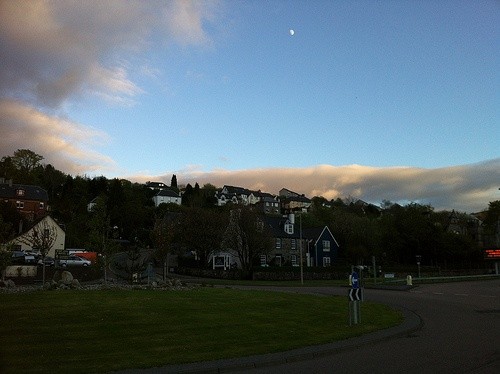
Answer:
[56,256,91,267]
[41,255,55,266]
